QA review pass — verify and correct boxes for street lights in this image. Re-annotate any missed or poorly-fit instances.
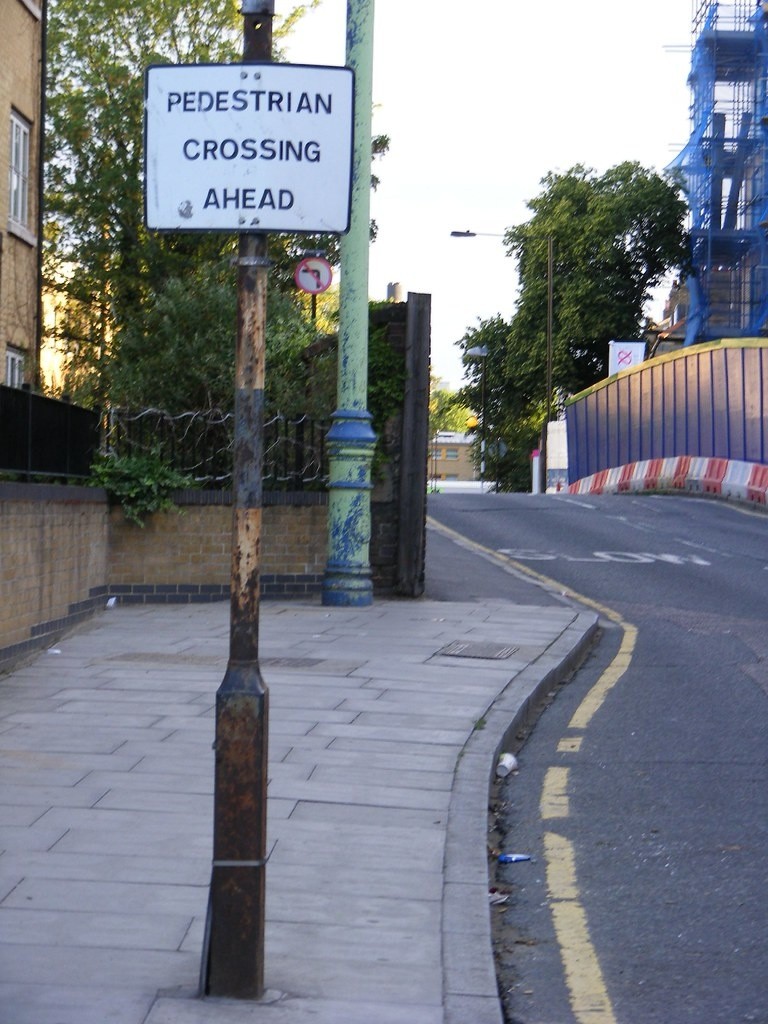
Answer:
[450,229,556,495]
[465,344,488,489]
